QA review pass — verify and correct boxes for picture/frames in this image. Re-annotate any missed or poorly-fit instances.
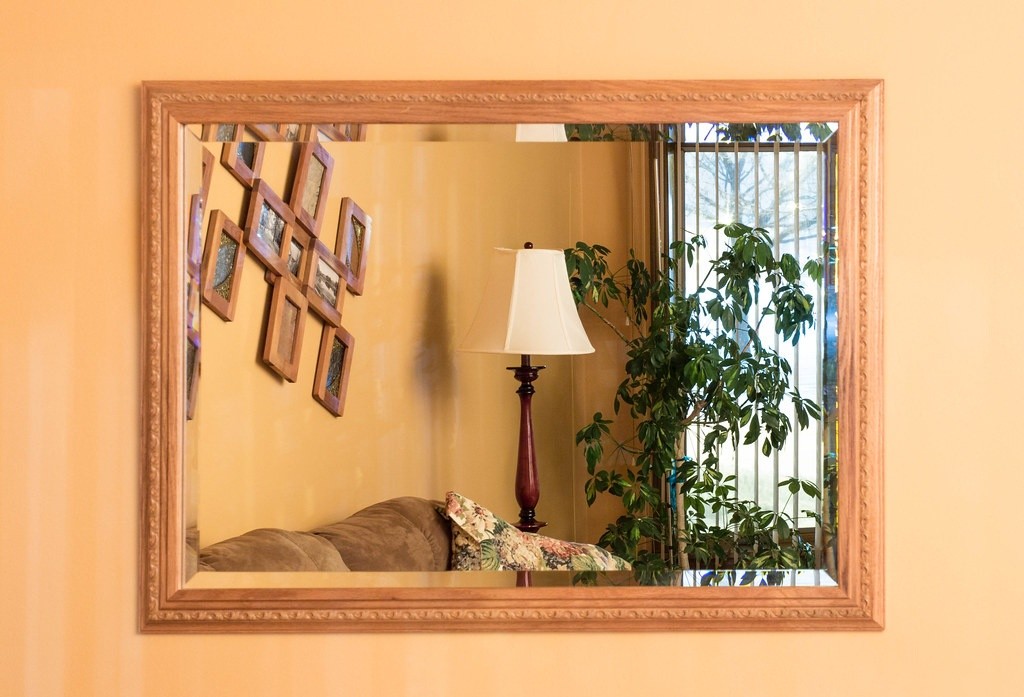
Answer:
[221,142,268,190]
[289,142,334,236]
[187,193,201,419]
[334,197,373,296]
[314,321,354,418]
[303,234,351,328]
[243,176,295,276]
[200,210,248,321]
[263,277,310,383]
[304,237,351,327]
[247,123,368,144]
[201,124,244,144]
[263,216,312,294]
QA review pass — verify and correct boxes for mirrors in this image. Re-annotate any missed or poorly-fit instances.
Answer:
[140,79,888,633]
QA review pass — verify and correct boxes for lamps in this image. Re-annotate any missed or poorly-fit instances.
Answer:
[457,240,596,533]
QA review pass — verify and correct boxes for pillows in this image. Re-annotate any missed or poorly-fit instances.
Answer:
[444,490,633,571]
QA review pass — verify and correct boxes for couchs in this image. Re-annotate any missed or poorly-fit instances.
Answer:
[198,496,460,572]
